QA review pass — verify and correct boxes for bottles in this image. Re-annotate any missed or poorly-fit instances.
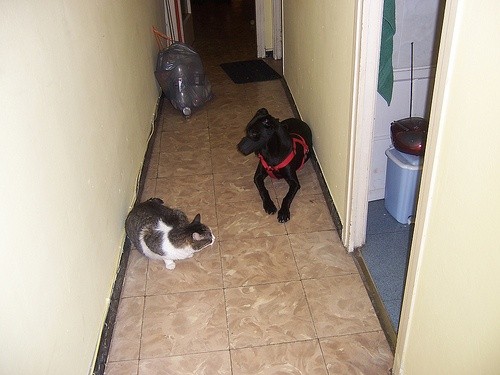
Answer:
[156,43,205,116]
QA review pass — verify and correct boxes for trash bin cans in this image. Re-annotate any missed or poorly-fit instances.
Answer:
[385,146,424,224]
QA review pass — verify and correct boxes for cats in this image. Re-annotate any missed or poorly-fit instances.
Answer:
[125,197,216,271]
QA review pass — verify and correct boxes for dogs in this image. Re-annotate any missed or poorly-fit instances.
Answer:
[236,107,314,223]
